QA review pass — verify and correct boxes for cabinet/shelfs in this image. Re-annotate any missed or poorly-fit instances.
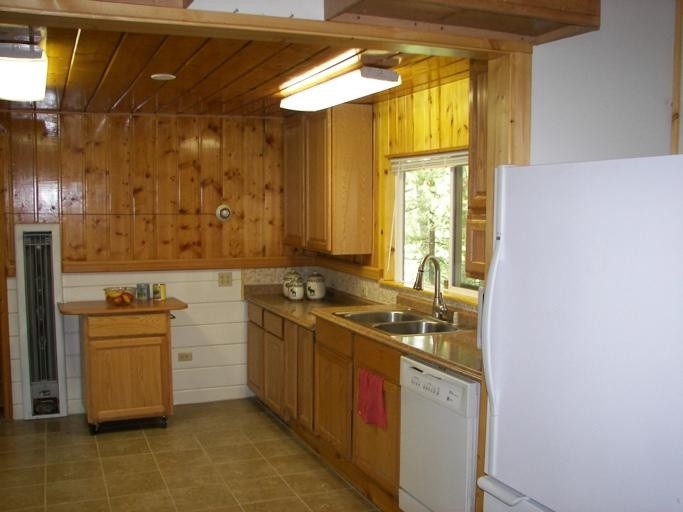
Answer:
[245,304,284,411]
[284,320,312,429]
[316,318,399,496]
[465,68,486,279]
[83,313,172,422]
[282,105,374,258]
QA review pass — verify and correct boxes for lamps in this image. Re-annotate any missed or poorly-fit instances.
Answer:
[0,44,47,103]
[280,67,402,112]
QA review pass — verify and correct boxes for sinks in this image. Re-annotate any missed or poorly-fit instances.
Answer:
[345,312,420,325]
[376,321,451,335]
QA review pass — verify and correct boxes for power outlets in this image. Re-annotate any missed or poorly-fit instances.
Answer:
[178,351,193,362]
[217,272,232,286]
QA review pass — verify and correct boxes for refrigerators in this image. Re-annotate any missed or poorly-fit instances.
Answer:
[476,153,683,512]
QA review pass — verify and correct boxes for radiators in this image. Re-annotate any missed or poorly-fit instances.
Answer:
[13,221,68,422]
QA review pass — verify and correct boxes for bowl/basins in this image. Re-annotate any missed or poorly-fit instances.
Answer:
[102,287,136,305]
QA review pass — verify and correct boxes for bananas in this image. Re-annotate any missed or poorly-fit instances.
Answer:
[104,286,127,297]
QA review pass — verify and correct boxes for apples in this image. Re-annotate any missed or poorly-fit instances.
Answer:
[121,293,134,302]
[113,297,122,304]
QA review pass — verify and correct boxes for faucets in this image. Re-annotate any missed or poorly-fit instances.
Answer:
[413,254,448,318]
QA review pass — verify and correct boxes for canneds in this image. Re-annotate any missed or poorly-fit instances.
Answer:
[137,283,150,301]
[154,283,167,299]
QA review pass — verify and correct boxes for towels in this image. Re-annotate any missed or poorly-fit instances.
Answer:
[357,368,387,431]
